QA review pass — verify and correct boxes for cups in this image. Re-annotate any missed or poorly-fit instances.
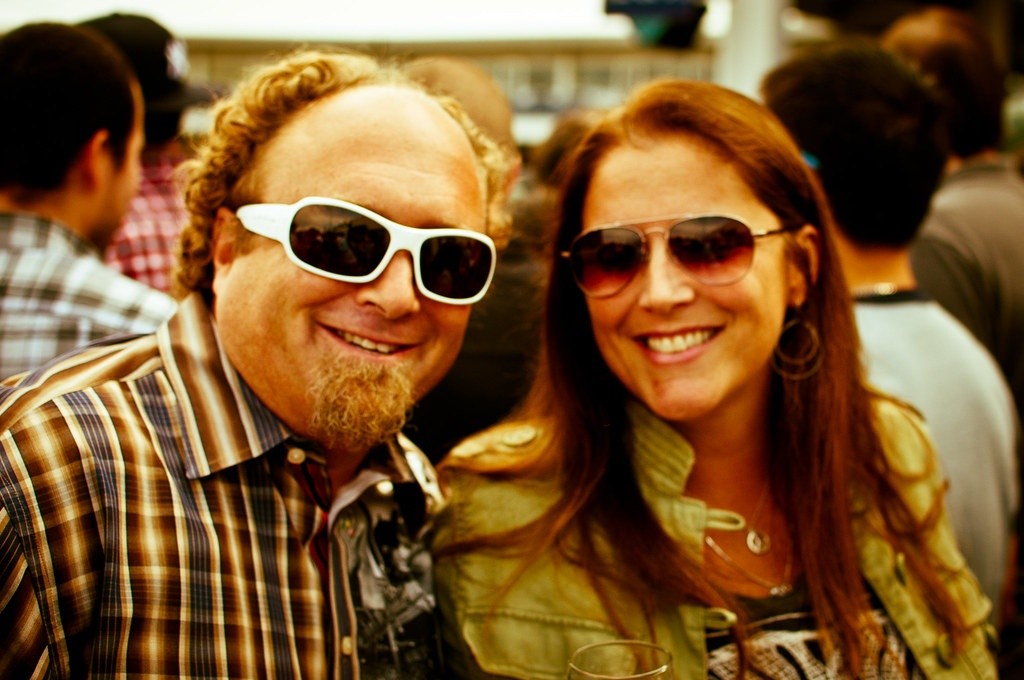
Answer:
[564,640,674,680]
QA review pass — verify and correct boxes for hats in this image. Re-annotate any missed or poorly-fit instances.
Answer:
[77,14,187,109]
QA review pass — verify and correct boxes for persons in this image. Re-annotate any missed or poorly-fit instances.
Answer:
[0,55,524,680]
[765,0,1024,680]
[425,76,1008,680]
[0,11,212,390]
[403,50,552,472]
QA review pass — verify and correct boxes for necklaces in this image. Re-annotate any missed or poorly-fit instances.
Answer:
[705,476,794,597]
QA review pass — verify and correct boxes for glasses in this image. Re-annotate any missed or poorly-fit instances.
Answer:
[561,216,791,300]
[237,196,497,305]
